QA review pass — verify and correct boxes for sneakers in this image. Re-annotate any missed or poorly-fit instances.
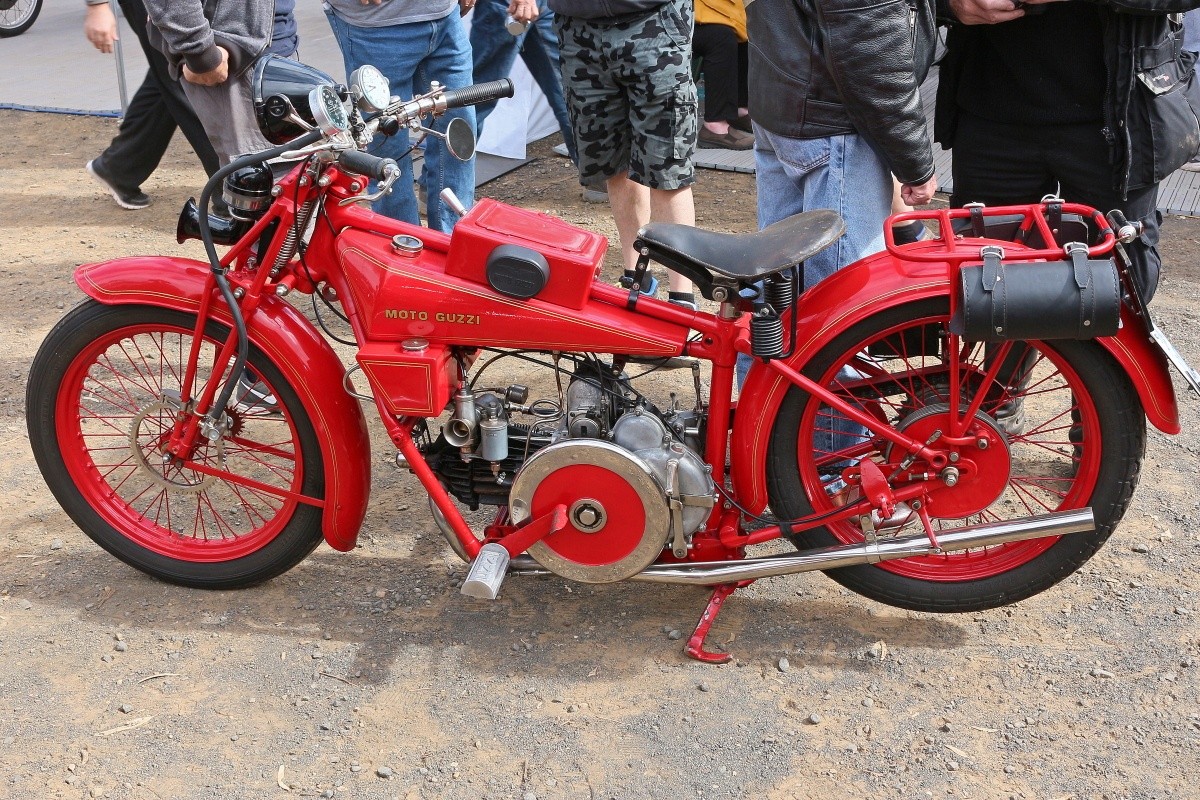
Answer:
[236,368,286,418]
[86,155,153,210]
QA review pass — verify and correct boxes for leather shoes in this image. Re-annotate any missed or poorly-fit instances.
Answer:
[829,484,917,536]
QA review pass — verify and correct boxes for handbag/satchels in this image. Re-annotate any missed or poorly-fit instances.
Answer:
[949,240,1124,344]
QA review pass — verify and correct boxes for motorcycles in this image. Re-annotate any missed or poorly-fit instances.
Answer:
[26,54,1200,665]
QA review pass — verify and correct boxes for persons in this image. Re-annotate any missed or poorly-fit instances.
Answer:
[469,0,610,202]
[738,1,937,531]
[140,0,301,214]
[546,0,699,313]
[323,0,478,232]
[693,0,755,150]
[932,0,1200,479]
[84,1,231,218]
[889,170,941,245]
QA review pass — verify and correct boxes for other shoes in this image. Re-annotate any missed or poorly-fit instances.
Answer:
[996,401,1026,438]
[580,181,609,205]
[697,123,756,150]
[611,276,658,300]
[728,113,754,134]
